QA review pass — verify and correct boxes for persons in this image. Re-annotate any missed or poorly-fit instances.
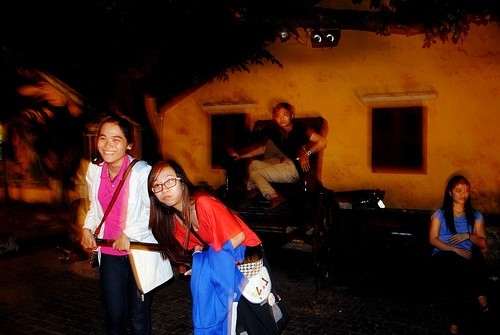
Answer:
[429,174,493,335]
[230,102,327,214]
[148,159,278,335]
[81,116,174,335]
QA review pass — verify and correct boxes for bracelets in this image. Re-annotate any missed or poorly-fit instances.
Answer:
[467,232,471,240]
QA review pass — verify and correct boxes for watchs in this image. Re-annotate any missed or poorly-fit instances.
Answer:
[307,151,311,156]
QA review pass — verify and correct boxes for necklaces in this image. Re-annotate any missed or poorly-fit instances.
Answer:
[108,173,118,183]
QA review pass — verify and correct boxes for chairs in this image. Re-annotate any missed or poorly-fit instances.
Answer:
[215,117,328,279]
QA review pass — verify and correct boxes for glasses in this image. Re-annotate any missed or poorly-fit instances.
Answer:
[151,178,181,193]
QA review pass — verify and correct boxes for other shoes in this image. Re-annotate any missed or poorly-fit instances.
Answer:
[247,187,260,200]
[266,195,287,211]
